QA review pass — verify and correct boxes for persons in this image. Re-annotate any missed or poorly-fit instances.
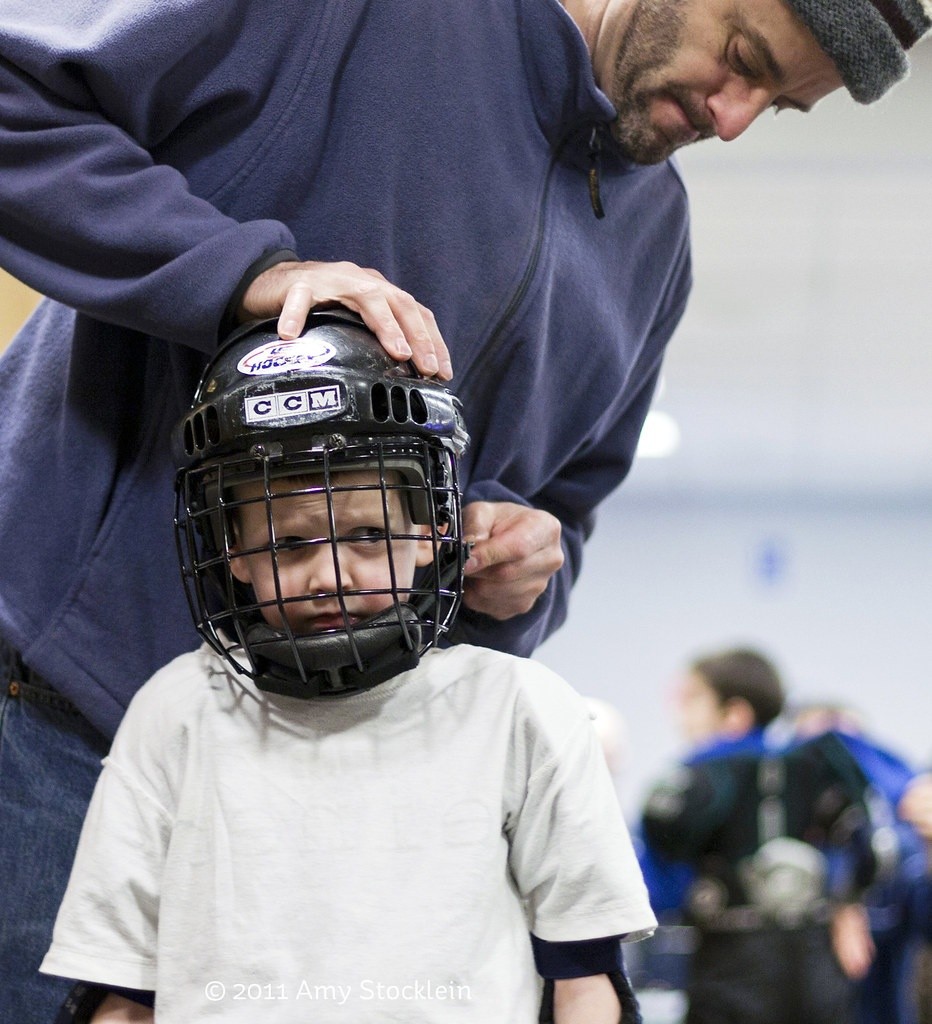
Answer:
[783,701,932,1024]
[37,310,659,1024]
[0,0,930,1024]
[628,650,879,1024]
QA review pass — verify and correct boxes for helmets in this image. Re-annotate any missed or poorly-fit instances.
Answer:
[173,308,475,700]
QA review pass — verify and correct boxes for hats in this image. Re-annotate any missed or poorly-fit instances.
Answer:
[788,0,932,106]
